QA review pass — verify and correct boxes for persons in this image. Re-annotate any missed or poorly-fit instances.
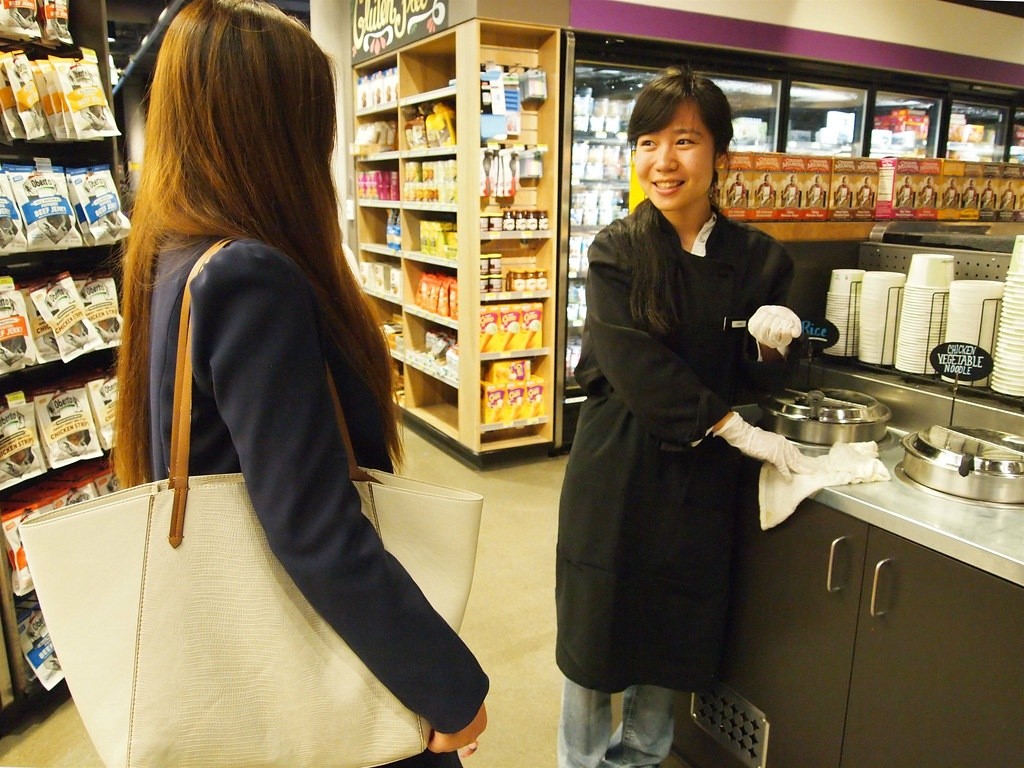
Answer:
[118,0,492,768]
[554,74,804,768]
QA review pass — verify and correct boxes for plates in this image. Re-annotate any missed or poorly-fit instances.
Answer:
[941,280,1004,387]
[858,271,906,365]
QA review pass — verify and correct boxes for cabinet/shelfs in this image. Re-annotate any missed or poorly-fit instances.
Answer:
[668,490,1024,768]
[347,17,564,470]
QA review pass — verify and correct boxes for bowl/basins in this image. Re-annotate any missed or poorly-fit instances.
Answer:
[895,254,954,375]
[822,268,864,356]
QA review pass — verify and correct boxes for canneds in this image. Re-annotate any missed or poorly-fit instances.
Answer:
[480,253,547,292]
[480,213,504,231]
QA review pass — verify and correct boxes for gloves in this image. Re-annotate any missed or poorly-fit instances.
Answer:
[748,305,802,349]
[713,411,816,481]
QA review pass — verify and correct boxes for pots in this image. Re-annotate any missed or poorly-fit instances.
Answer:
[756,385,892,447]
[900,425,1024,504]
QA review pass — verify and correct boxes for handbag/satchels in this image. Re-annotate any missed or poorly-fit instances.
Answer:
[18,239,483,768]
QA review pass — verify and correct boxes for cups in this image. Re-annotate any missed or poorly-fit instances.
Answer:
[990,234,1024,397]
